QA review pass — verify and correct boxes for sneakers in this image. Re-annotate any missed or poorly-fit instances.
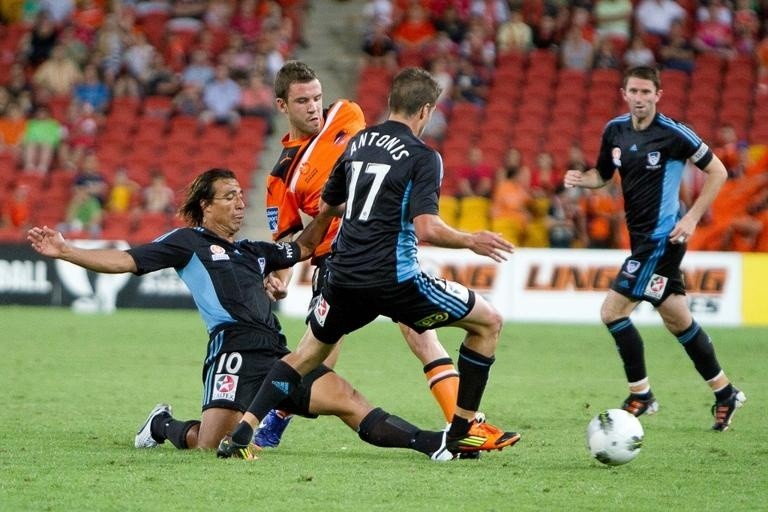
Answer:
[429,411,487,463]
[216,435,264,459]
[621,387,660,417]
[712,385,746,433]
[458,449,481,461]
[253,409,293,452]
[446,413,521,450]
[134,402,173,450]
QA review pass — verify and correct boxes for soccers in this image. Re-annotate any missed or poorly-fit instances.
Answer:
[586,409,645,466]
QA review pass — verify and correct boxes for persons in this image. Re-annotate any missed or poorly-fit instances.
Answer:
[23,163,488,465]
[247,59,516,450]
[455,123,746,221]
[213,66,506,460]
[0,1,293,171]
[422,35,491,113]
[558,63,749,433]
[68,166,175,233]
[358,0,766,71]
